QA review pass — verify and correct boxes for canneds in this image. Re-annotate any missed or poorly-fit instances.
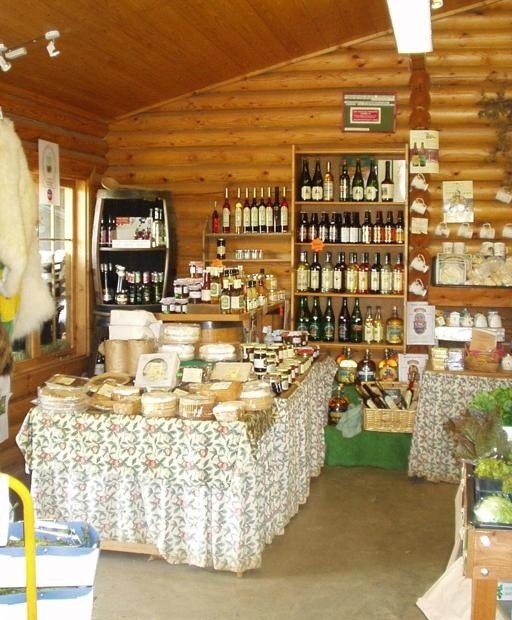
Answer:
[217,238,226,260]
[265,273,286,305]
[235,249,264,260]
[240,330,314,395]
[160,261,205,315]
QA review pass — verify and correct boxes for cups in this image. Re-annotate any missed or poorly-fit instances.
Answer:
[408,174,511,299]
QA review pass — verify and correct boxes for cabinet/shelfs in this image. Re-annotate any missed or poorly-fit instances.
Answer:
[143,296,287,355]
[91,180,178,331]
[194,221,295,315]
[287,142,412,399]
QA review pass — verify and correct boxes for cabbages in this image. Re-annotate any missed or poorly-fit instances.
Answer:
[473,495,512,524]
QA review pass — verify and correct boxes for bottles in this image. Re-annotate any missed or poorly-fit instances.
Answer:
[212,186,289,234]
[98,206,164,303]
[433,310,502,328]
[298,157,403,381]
[419,142,425,167]
[405,372,418,410]
[93,338,107,374]
[502,352,512,372]
[235,249,264,259]
[328,383,347,424]
[355,382,400,410]
[165,263,286,315]
[412,141,418,166]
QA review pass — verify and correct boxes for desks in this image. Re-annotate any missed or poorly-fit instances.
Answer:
[19,333,346,575]
[403,359,512,483]
[437,446,512,620]
